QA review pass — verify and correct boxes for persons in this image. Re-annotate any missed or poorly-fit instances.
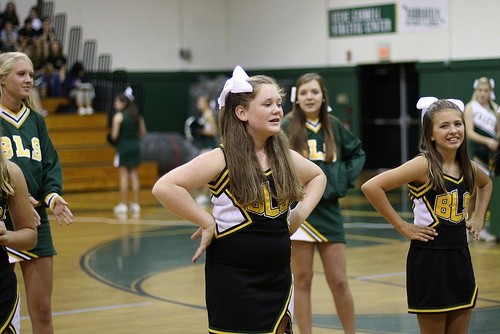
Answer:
[0,2,66,117]
[0,52,73,334]
[281,72,365,334]
[464,77,500,242]
[107,86,146,213]
[63,62,95,115]
[0,141,38,334]
[153,66,327,334]
[361,97,492,334]
[185,94,217,204]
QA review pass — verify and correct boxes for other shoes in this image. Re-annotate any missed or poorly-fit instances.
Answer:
[112,203,128,213]
[477,229,495,242]
[130,202,141,212]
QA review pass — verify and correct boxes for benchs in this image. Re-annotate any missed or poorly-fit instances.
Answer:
[41,98,158,193]
